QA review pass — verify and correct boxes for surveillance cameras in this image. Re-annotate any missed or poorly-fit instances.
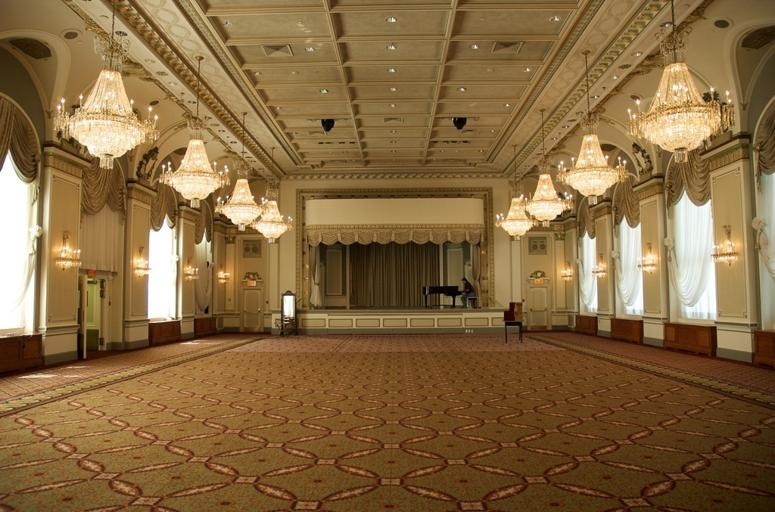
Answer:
[453,118,466,130]
[321,119,334,132]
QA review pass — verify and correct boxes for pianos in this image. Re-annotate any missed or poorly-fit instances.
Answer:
[422,286,462,308]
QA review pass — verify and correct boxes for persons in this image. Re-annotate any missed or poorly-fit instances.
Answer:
[460,278,475,309]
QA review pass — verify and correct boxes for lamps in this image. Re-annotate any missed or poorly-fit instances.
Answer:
[707,223,746,268]
[250,147,289,244]
[555,50,627,205]
[495,144,539,240]
[627,1,736,164]
[215,112,264,231]
[52,1,161,171]
[524,108,573,229]
[51,232,82,271]
[158,56,231,208]
